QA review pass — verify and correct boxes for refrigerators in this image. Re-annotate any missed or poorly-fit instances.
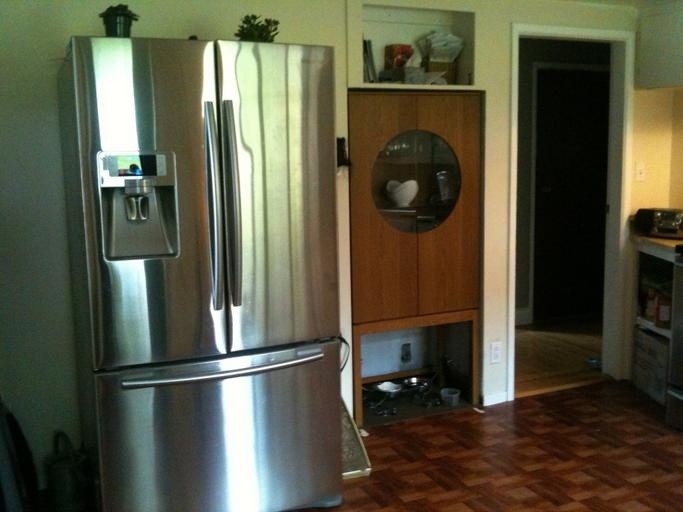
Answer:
[56,38,342,511]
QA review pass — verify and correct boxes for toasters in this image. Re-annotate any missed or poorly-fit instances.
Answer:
[632,205,682,238]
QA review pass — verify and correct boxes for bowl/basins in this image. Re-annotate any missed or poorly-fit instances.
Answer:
[440,389,460,407]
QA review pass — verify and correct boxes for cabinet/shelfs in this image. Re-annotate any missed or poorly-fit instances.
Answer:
[345,92,484,428]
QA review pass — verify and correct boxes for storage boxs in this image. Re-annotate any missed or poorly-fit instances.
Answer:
[625,324,669,405]
